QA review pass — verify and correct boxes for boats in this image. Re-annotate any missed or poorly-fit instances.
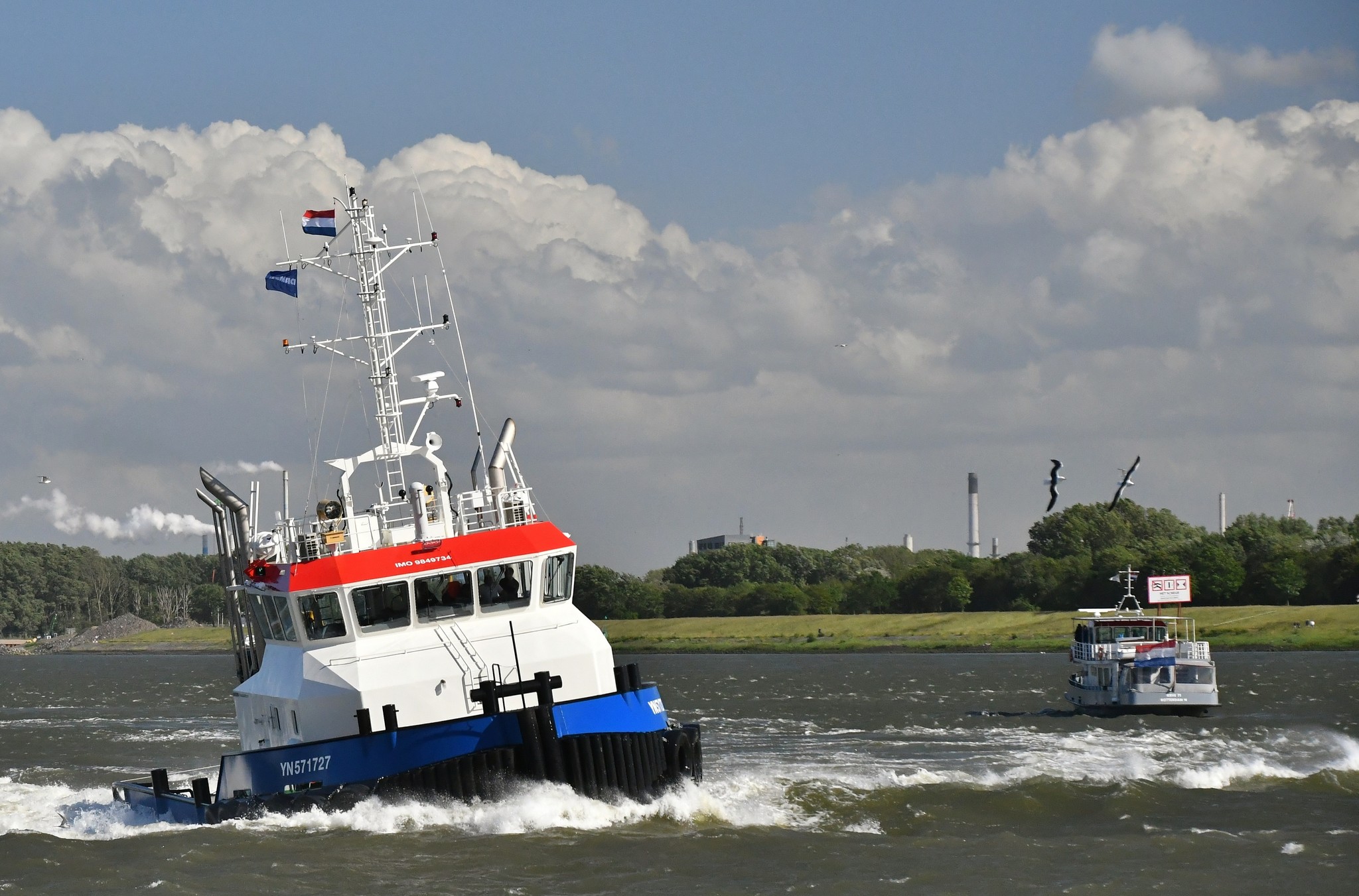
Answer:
[63,183,703,838]
[1071,563,1221,719]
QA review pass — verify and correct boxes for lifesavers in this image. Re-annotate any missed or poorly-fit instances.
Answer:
[1098,646,1104,661]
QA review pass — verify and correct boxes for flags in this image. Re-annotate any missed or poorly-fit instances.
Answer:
[1129,572,1138,580]
[1134,639,1177,667]
[302,208,337,238]
[265,268,298,299]
[1109,574,1121,583]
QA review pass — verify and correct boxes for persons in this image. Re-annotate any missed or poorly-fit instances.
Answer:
[1074,622,1089,644]
[479,575,507,605]
[499,567,520,604]
[416,582,441,611]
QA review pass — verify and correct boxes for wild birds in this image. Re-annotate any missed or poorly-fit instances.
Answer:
[1042,457,1067,513]
[835,344,848,348]
[36,475,52,484]
[1107,454,1142,513]
[52,811,72,830]
[77,358,84,361]
